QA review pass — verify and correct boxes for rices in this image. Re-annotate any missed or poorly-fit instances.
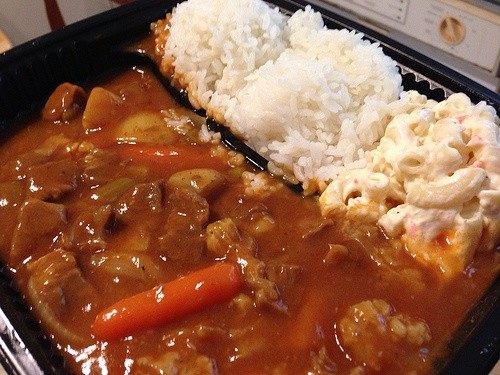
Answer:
[150,0,413,196]
